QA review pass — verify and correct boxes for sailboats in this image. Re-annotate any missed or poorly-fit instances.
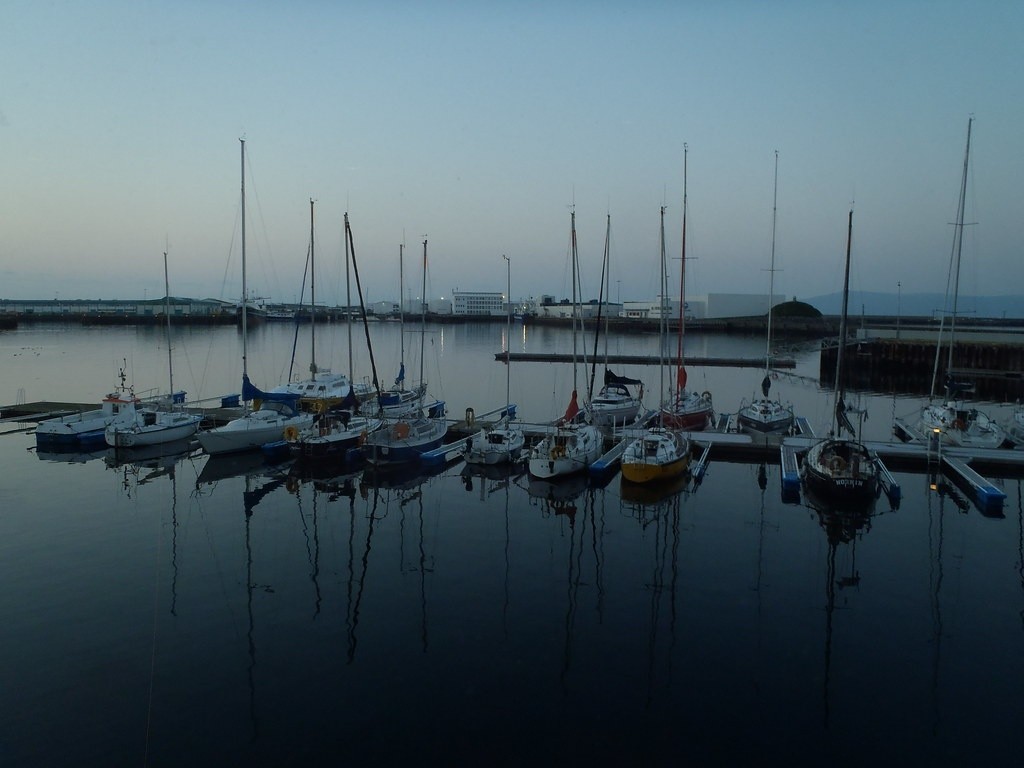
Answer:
[33,111,1008,506]
[37,443,692,744]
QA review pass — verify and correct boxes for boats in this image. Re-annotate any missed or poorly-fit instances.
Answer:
[385,316,401,322]
[354,316,380,321]
[235,296,267,330]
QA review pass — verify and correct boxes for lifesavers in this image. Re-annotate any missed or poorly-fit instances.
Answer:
[830,456,845,471]
[551,446,565,460]
[701,391,711,400]
[285,426,297,440]
